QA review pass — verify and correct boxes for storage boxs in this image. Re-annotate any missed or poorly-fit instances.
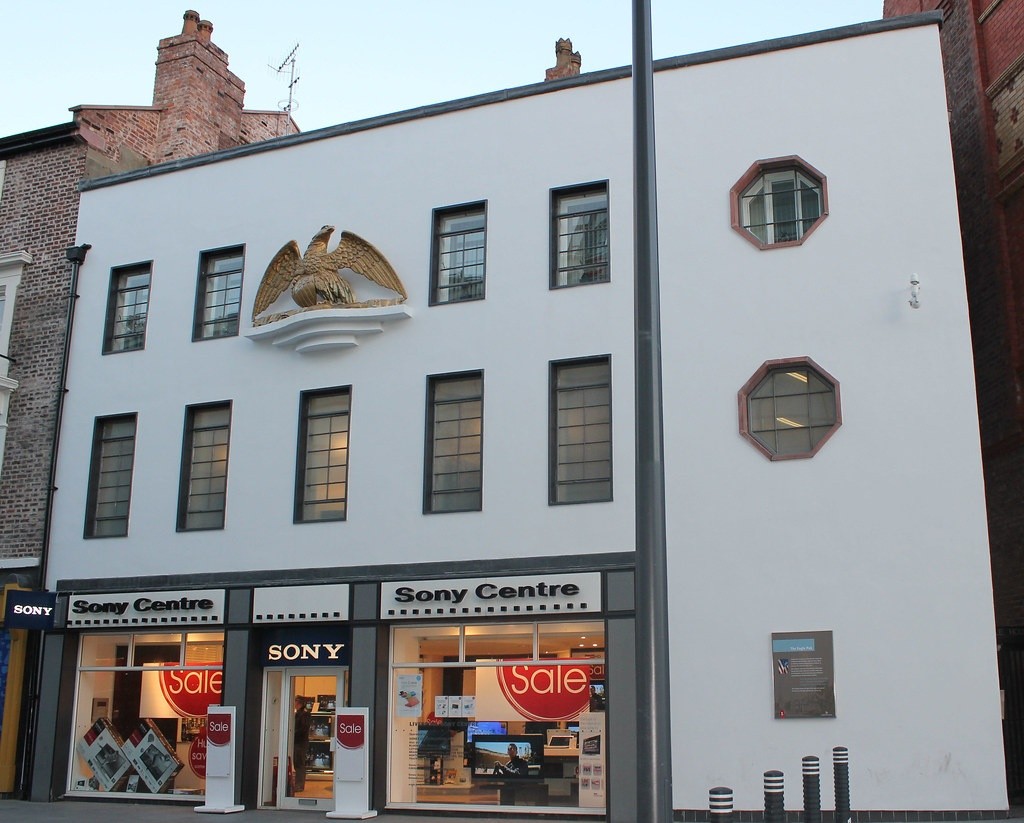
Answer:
[121,713,186,796]
[173,788,205,796]
[124,774,140,794]
[77,715,132,794]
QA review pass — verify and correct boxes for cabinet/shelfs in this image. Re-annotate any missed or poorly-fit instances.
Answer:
[305,711,334,781]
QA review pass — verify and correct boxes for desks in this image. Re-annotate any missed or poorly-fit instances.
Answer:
[544,747,579,764]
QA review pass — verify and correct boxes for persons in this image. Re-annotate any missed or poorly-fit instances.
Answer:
[589,686,605,710]
[493,743,528,776]
[293,694,311,791]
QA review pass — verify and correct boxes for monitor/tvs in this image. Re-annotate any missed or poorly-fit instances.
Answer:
[308,716,335,740]
[305,740,335,774]
[566,722,580,734]
[589,684,605,701]
[471,734,544,784]
[464,720,508,752]
